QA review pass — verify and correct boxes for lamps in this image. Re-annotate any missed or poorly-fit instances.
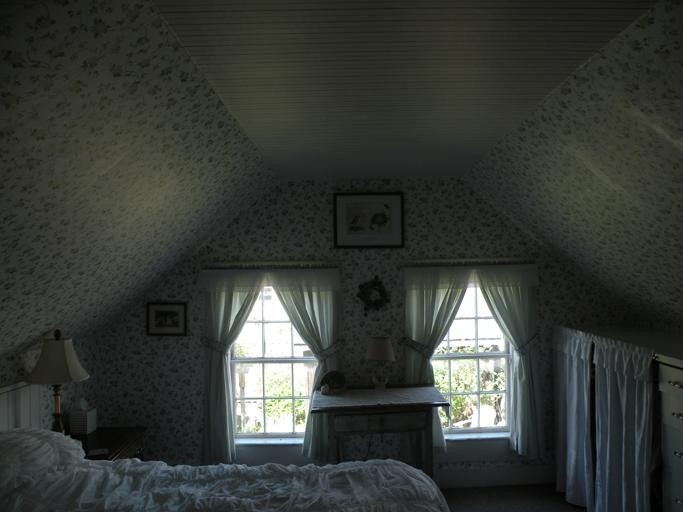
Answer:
[367,335,395,391]
[23,329,90,433]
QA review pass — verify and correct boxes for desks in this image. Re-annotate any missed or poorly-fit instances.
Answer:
[310,384,449,478]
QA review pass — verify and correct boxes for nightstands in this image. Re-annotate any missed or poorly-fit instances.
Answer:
[82,427,151,462]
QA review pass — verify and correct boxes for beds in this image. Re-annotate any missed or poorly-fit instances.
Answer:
[0,380,451,512]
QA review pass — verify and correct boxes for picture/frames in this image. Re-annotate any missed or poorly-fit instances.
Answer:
[333,191,405,249]
[146,301,188,336]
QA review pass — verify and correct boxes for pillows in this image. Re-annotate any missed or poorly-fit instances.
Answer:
[0,426,85,497]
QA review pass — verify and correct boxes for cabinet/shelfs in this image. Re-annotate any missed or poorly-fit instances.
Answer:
[649,352,683,512]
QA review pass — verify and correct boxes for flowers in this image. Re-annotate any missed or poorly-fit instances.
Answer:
[356,275,390,317]
